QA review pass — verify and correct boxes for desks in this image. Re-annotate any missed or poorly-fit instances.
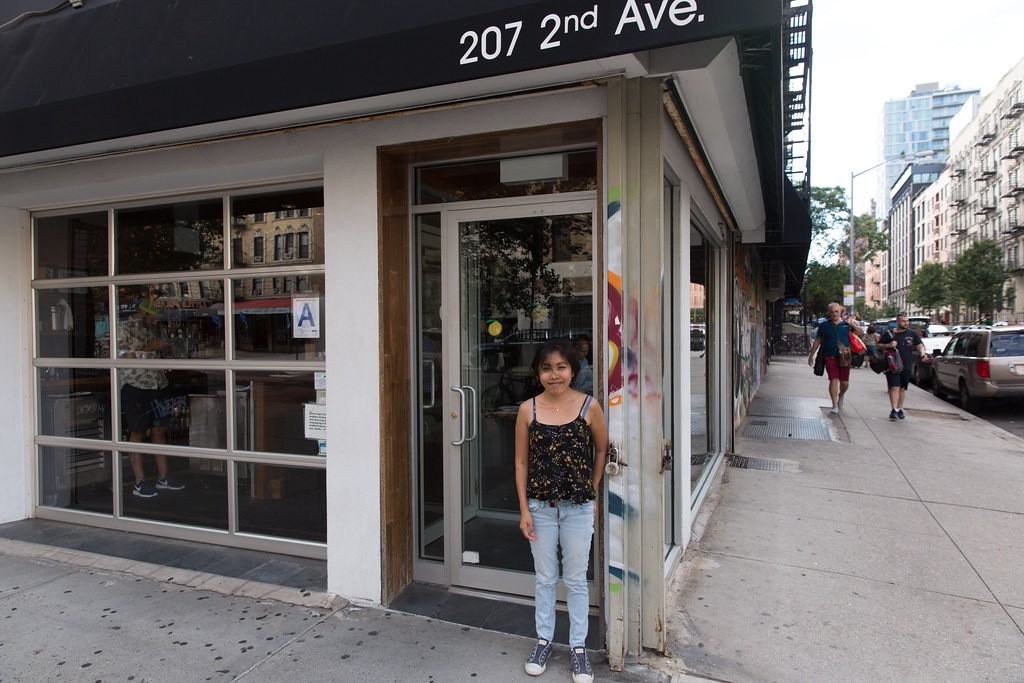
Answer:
[224,372,316,500]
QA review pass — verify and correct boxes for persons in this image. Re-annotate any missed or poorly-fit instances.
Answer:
[119,299,186,498]
[809,303,864,413]
[481,319,505,419]
[514,336,607,683]
[519,339,594,401]
[877,314,929,421]
[843,314,880,368]
[423,336,442,424]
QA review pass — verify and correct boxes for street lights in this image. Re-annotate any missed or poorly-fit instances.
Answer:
[850,149,935,319]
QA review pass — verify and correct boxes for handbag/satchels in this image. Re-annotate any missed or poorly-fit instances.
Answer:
[886,329,904,374]
[848,330,867,356]
[869,349,890,375]
[813,348,826,377]
[837,342,852,368]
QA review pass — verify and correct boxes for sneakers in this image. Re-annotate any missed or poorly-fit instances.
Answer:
[131,480,158,498]
[155,477,185,490]
[569,647,594,683]
[524,642,553,676]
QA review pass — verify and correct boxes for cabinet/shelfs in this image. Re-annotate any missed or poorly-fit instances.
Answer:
[40,264,97,373]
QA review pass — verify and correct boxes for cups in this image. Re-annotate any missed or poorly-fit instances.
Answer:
[45,305,65,330]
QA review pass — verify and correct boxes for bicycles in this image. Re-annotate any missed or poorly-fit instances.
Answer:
[776,334,812,356]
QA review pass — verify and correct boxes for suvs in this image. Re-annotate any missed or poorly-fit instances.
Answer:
[932,322,1024,411]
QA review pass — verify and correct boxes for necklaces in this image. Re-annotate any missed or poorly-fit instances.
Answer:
[553,408,560,416]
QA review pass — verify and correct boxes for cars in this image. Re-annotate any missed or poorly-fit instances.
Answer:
[422,330,444,390]
[469,329,590,372]
[811,318,827,326]
[858,316,1018,388]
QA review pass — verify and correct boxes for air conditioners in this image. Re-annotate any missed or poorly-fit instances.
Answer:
[284,254,292,259]
[255,256,262,261]
[273,288,278,293]
[254,290,261,295]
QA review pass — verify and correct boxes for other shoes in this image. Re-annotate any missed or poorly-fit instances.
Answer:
[888,409,898,418]
[830,405,838,412]
[838,394,843,407]
[864,364,868,368]
[897,408,904,419]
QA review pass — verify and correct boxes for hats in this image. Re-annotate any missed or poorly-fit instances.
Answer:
[138,299,165,318]
